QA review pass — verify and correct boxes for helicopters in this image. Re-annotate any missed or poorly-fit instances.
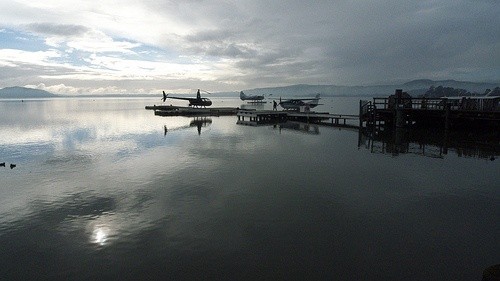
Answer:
[279,92,324,111]
[240,91,268,104]
[161,89,212,109]
